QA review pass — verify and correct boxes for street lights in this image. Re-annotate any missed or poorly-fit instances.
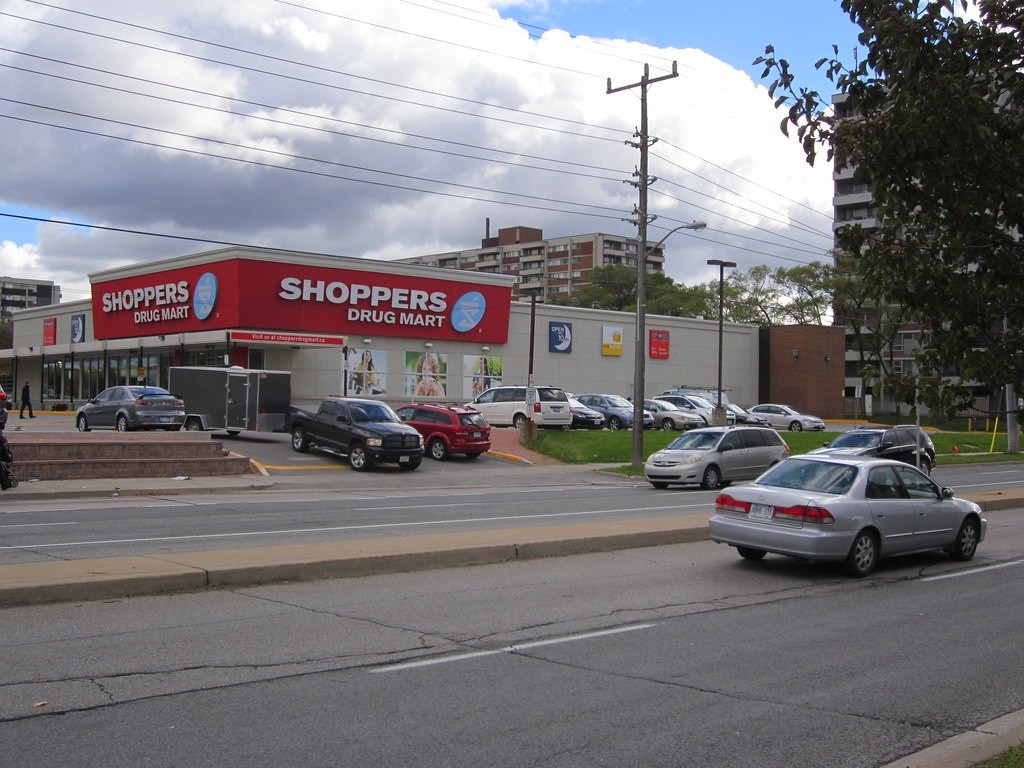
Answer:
[629,220,707,474]
[707,258,738,429]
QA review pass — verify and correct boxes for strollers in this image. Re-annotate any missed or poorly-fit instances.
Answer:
[0,437,19,486]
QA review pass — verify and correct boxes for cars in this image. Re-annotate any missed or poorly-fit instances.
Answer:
[645,425,790,493]
[395,400,492,461]
[707,453,987,578]
[563,387,749,432]
[746,401,826,433]
[73,383,187,434]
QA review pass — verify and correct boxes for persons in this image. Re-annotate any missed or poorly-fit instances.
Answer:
[414,354,445,397]
[19,381,36,419]
[472,357,491,397]
[354,350,375,394]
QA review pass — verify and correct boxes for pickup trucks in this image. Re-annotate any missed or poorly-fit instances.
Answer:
[284,392,424,472]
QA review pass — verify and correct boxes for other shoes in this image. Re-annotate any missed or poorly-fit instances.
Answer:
[20,416,26,419]
[30,416,36,418]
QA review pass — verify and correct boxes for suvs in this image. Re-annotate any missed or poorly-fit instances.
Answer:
[462,386,573,432]
[806,422,937,481]
